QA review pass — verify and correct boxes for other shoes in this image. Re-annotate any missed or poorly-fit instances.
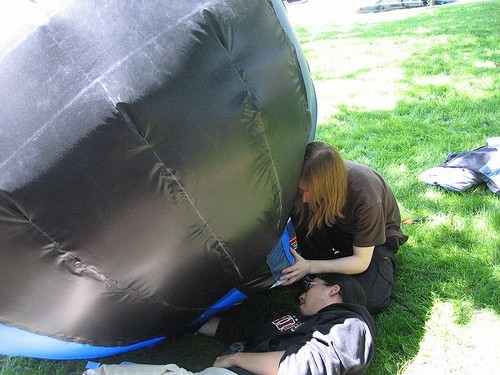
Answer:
[304,277,313,284]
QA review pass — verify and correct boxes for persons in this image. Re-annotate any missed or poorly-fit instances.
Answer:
[195,273,377,375]
[277,140,409,313]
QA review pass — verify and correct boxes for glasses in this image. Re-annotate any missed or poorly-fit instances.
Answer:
[306,282,333,289]
